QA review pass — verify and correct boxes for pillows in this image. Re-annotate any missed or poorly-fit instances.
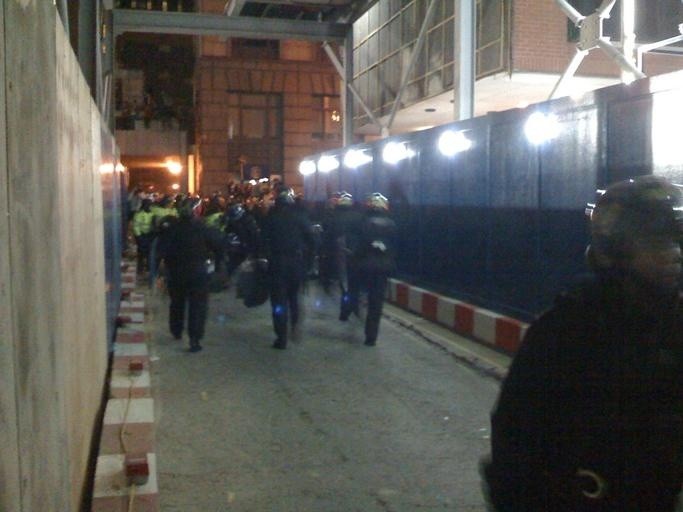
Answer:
[278,188,295,205]
[366,192,389,211]
[336,190,353,207]
[583,176,682,274]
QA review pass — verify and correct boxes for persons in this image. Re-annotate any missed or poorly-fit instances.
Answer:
[124,174,364,291]
[340,192,402,345]
[478,175,683,512]
[155,189,239,349]
